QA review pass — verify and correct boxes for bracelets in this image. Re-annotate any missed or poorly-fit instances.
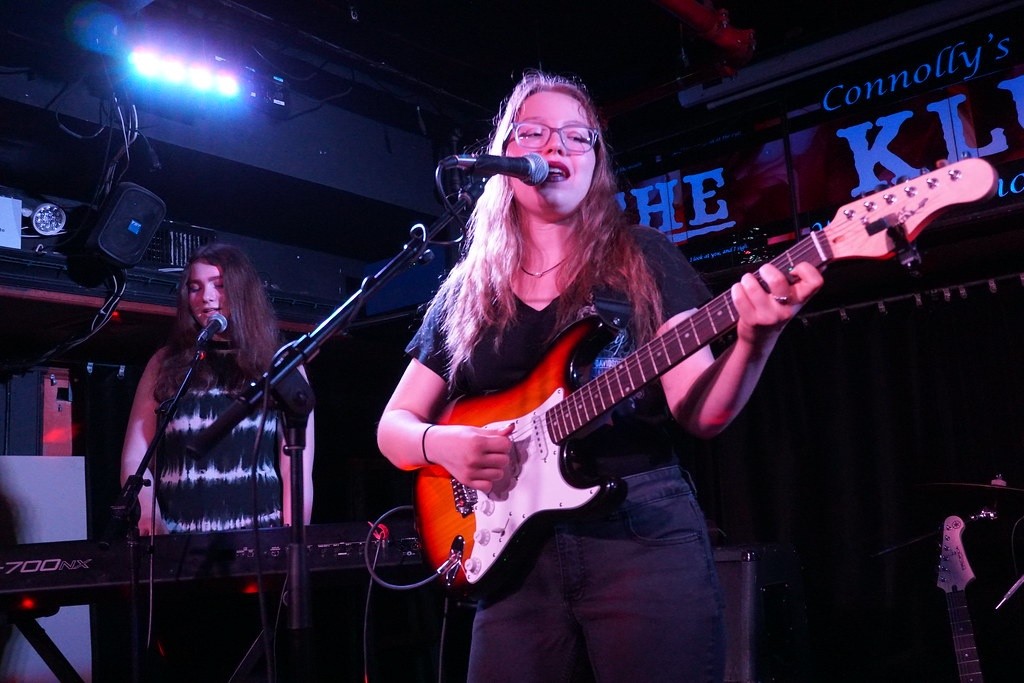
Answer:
[422,424,437,463]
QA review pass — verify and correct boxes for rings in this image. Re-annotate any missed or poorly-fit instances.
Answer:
[774,295,791,304]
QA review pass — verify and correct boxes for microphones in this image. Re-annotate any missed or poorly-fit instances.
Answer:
[197,314,227,343]
[440,152,549,186]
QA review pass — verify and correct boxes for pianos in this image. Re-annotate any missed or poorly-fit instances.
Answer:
[0,513,431,609]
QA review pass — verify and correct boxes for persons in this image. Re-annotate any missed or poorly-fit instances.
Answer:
[377,76,824,683]
[119,241,316,683]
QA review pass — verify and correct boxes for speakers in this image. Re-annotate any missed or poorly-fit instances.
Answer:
[707,542,809,683]
[83,182,167,269]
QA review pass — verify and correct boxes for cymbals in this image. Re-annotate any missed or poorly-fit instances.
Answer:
[924,479,1024,498]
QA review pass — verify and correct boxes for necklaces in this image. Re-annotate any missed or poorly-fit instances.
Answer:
[515,246,576,277]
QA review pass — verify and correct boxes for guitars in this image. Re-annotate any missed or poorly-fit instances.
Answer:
[414,158,999,593]
[933,514,984,683]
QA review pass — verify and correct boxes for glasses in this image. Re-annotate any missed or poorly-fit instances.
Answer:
[504,119,598,152]
